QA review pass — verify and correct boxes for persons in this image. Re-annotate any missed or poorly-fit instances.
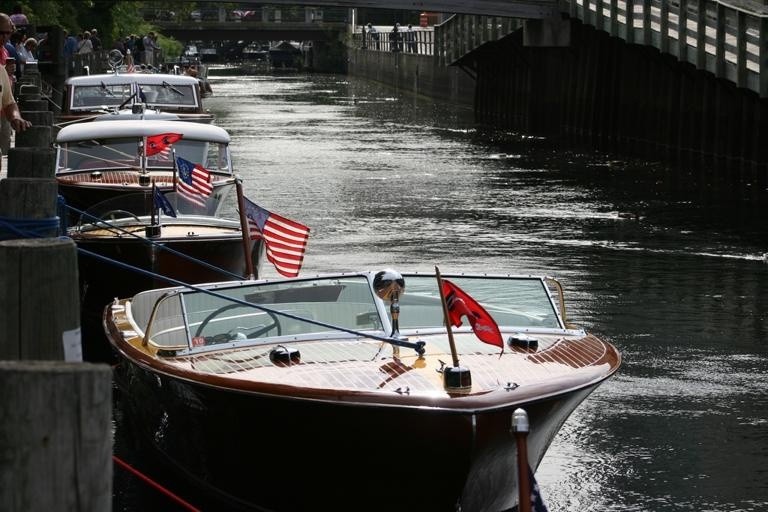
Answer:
[406,24,418,53]
[366,23,380,50]
[389,22,403,52]
[0,12,162,169]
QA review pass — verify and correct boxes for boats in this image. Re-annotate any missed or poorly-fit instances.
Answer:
[56,60,216,123]
[100,263,623,512]
[50,103,236,223]
[52,176,267,296]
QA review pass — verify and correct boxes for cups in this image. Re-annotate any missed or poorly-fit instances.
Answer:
[7,58,16,72]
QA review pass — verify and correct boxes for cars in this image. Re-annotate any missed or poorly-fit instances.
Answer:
[140,7,263,24]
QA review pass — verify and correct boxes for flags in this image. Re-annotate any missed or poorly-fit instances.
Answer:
[441,279,504,358]
[175,156,214,208]
[243,196,310,277]
[146,133,184,157]
